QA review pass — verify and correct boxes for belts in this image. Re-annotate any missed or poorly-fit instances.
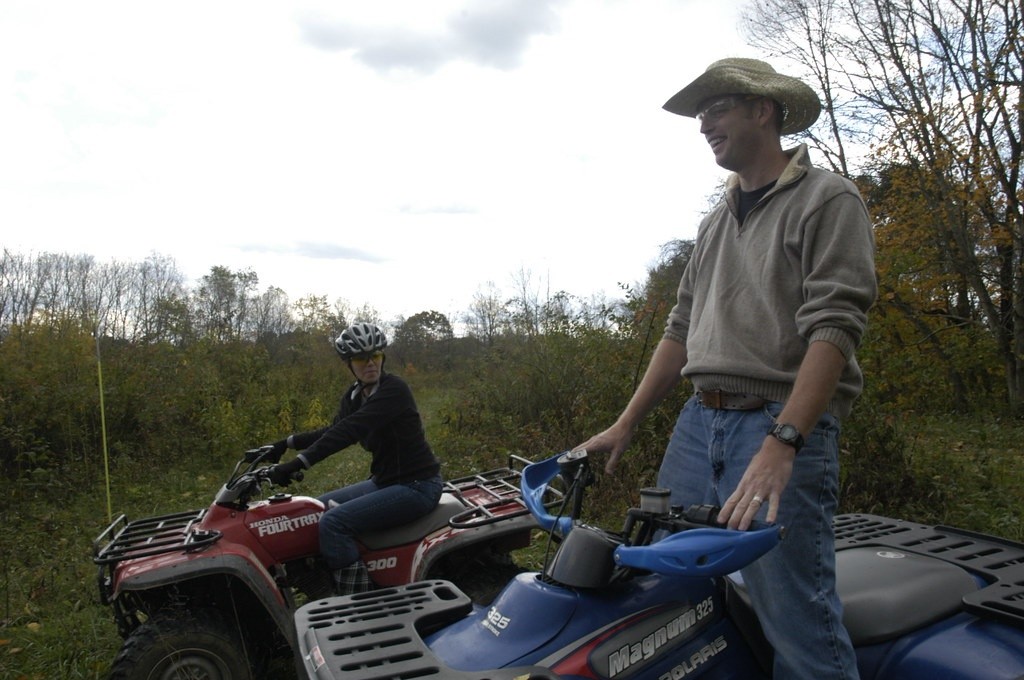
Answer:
[697,389,768,410]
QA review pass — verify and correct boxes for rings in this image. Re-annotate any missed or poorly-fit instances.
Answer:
[753,497,763,504]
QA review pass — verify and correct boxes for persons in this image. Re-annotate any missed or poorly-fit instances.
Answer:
[572,59,877,680]
[266,322,443,623]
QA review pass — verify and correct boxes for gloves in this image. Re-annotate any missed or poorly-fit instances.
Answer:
[267,457,304,488]
[262,439,288,464]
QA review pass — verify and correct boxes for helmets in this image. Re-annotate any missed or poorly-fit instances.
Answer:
[333,322,387,360]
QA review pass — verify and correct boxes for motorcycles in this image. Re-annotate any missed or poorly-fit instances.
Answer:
[293,449,1024,680]
[89,444,570,680]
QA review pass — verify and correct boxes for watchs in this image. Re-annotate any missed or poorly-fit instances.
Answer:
[767,423,804,454]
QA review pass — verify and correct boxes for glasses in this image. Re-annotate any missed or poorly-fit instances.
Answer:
[350,350,383,365]
[696,95,763,126]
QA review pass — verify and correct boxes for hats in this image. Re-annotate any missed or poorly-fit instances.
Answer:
[661,58,822,135]
